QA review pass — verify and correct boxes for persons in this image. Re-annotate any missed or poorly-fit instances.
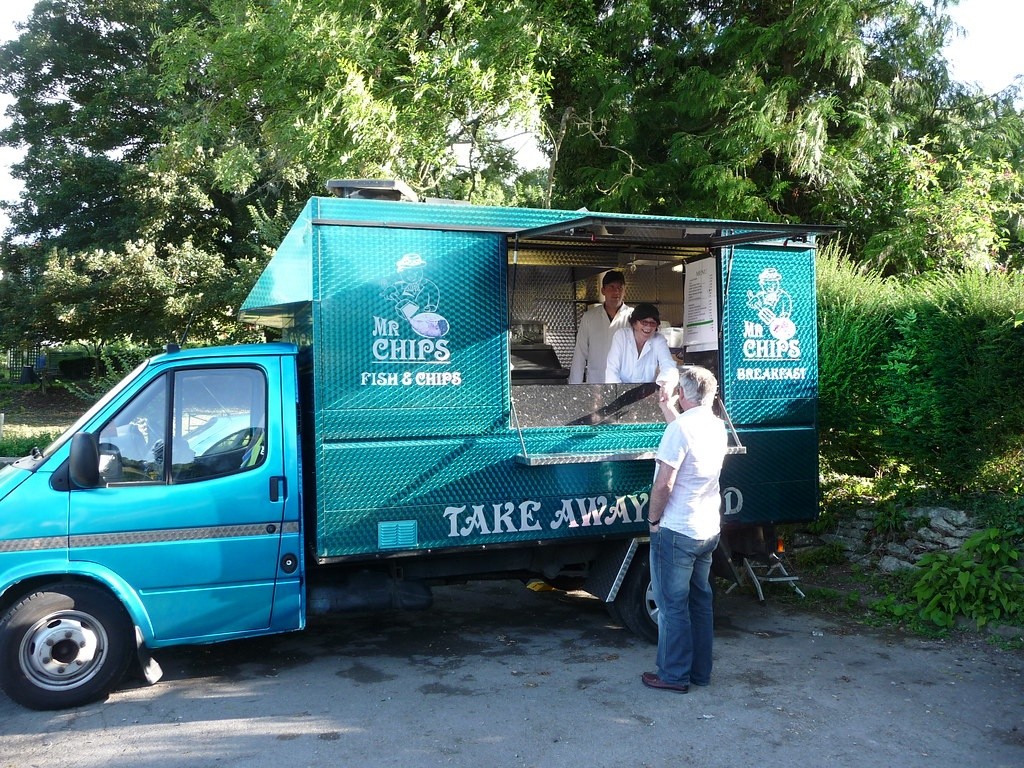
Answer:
[605,302,679,400]
[642,365,727,694]
[568,270,636,384]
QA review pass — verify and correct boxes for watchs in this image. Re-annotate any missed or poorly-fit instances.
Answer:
[647,518,660,525]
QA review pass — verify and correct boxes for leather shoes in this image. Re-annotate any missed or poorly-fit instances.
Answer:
[642,672,689,693]
[688,676,709,686]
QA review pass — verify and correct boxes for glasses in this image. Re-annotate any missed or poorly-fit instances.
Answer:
[639,320,659,327]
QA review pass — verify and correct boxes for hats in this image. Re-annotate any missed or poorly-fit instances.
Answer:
[632,304,659,320]
[603,271,624,284]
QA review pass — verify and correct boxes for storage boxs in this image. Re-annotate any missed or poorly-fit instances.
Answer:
[508,320,549,345]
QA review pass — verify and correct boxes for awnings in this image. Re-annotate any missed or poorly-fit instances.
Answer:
[508,215,847,337]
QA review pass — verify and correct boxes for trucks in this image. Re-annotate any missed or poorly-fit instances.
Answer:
[0,179,848,711]
